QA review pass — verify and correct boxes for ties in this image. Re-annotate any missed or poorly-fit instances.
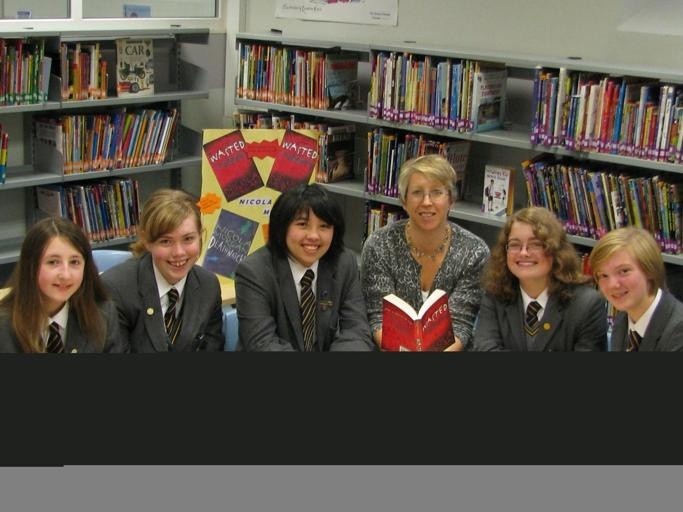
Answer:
[524,301,541,336]
[165,289,183,345]
[300,270,320,352]
[625,329,641,352]
[46,322,65,354]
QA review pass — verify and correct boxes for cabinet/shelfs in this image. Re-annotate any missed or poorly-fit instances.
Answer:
[233,32,683,276]
[0,20,211,281]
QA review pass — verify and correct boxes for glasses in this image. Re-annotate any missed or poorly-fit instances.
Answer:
[406,188,448,201]
[506,240,547,253]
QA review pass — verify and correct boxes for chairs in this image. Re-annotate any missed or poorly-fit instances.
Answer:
[89,251,134,274]
[222,310,241,353]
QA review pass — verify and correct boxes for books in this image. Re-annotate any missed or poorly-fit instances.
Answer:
[36,180,142,243]
[34,110,179,174]
[238,116,682,259]
[380,287,454,351]
[0,123,9,183]
[236,34,682,166]
[0,32,154,107]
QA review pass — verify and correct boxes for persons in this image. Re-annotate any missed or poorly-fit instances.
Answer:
[587,227,682,351]
[359,155,491,353]
[472,205,608,350]
[92,187,226,352]
[232,182,374,352]
[0,215,123,355]
